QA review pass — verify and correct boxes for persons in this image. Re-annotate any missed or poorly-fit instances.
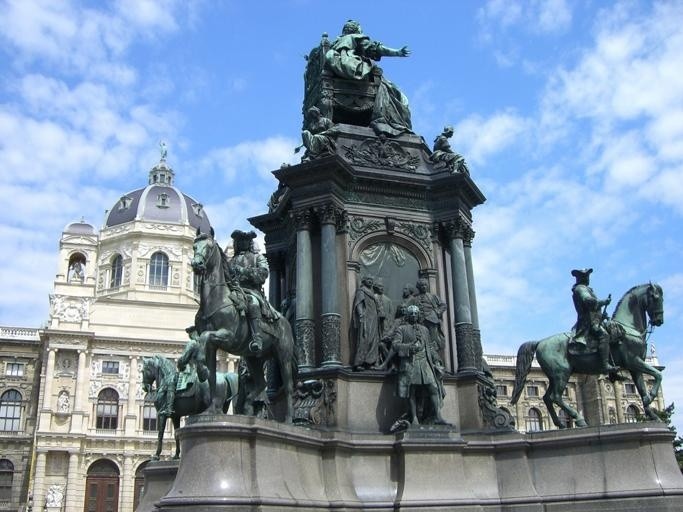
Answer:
[160,327,208,419]
[53,295,63,315]
[352,276,455,426]
[160,140,168,159]
[230,229,268,354]
[57,392,70,412]
[302,20,465,175]
[83,298,89,317]
[46,485,63,507]
[570,269,629,381]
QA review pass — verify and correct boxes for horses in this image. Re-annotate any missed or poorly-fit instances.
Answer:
[177,226,294,425]
[140,355,231,462]
[510,281,663,429]
